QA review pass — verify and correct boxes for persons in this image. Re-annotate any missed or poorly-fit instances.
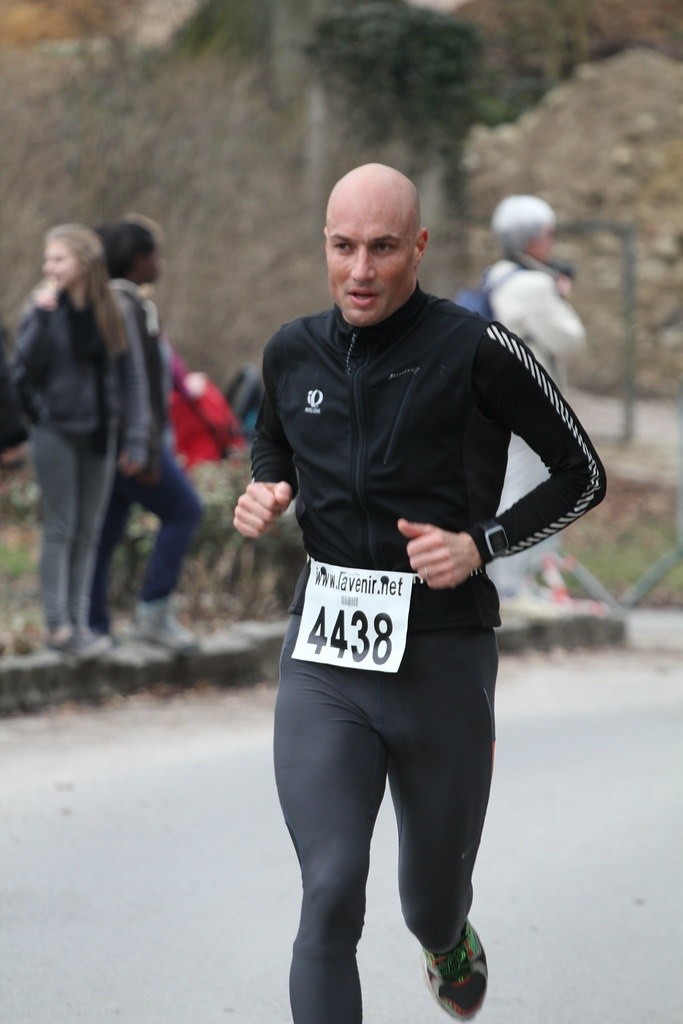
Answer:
[0,213,205,655]
[232,164,606,1024]
[482,195,586,599]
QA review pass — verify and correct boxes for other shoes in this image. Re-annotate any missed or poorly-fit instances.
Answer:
[43,633,113,659]
[129,601,194,649]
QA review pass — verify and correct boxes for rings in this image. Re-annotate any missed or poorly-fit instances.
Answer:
[424,565,430,578]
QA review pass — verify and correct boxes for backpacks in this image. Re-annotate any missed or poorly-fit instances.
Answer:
[454,265,524,319]
[165,357,246,471]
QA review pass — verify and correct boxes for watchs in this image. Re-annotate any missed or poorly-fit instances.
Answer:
[477,520,510,557]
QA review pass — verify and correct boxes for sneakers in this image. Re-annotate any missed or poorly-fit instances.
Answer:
[423,918,487,1020]
